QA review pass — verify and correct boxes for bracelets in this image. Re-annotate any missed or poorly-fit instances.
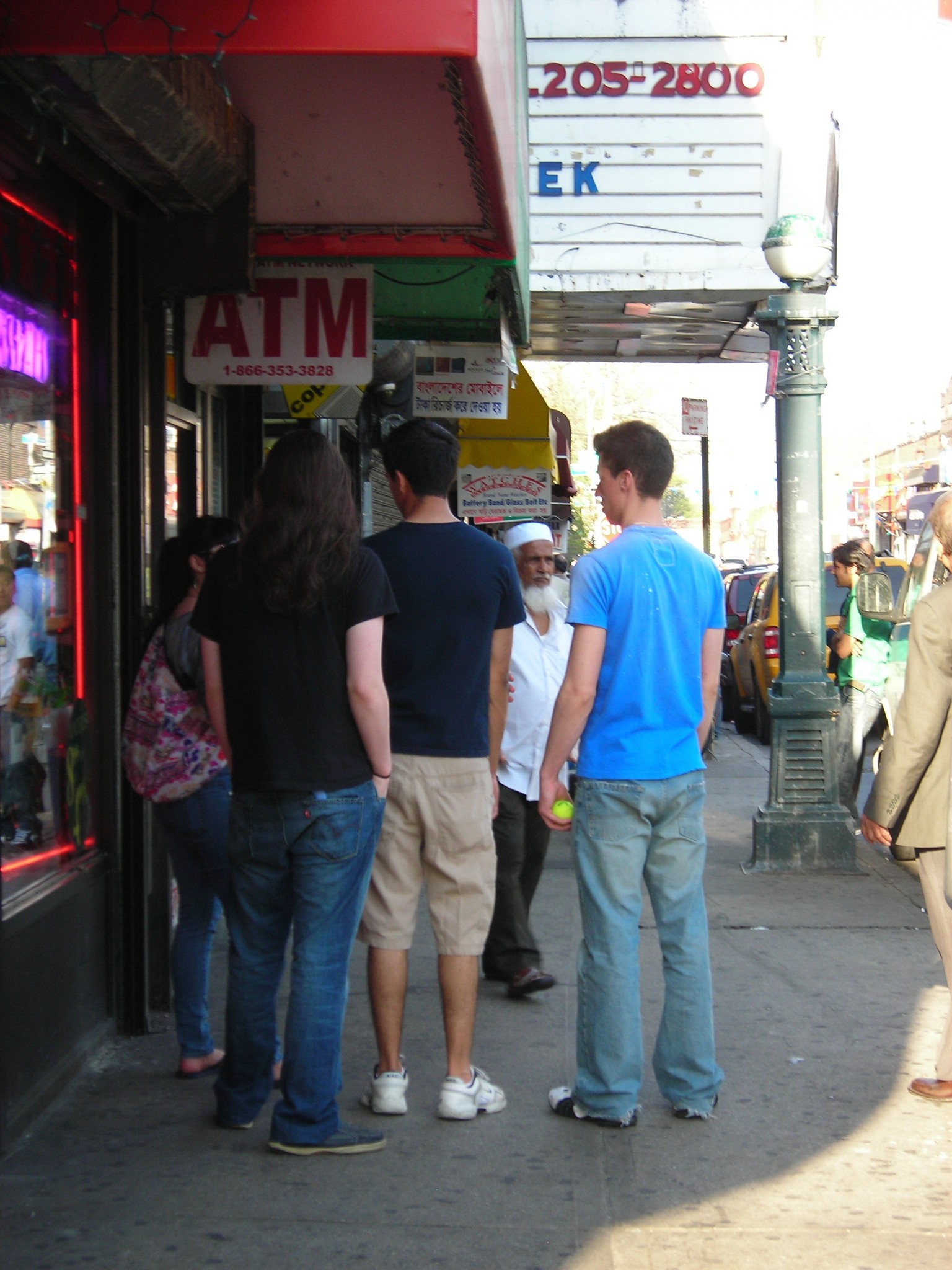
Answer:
[372,770,391,779]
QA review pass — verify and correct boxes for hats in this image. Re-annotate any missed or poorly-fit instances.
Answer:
[503,521,554,548]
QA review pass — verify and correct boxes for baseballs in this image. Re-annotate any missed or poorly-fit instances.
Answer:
[552,799,574,819]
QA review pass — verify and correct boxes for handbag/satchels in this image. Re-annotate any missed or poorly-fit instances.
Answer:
[119,625,229,804]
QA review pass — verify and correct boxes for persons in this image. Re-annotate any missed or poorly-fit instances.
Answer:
[145,515,286,1084]
[192,428,397,1153]
[860,491,952,1103]
[828,538,893,835]
[482,523,576,995]
[0,531,57,850]
[356,417,528,1122]
[537,421,726,1129]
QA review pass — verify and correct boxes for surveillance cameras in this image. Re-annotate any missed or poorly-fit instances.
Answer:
[372,383,396,399]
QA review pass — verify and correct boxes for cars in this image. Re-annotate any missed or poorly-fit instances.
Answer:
[715,479,951,775]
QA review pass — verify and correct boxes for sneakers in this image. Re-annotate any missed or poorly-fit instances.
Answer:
[436,1064,506,1120]
[358,1053,409,1115]
[220,1111,255,1131]
[267,1124,385,1158]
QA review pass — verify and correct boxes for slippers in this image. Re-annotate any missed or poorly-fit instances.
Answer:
[268,1059,287,1088]
[175,1049,227,1080]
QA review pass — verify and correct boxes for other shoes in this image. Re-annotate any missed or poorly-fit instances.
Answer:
[907,1077,952,1104]
[674,1091,720,1120]
[482,965,509,982]
[507,965,556,997]
[548,1084,639,1127]
